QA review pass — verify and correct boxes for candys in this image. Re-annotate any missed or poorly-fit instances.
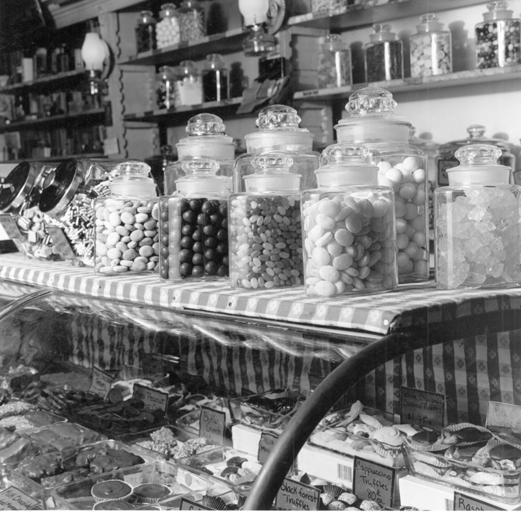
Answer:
[17,0,520,299]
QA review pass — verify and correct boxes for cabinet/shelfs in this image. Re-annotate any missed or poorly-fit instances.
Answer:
[0,0,521,512]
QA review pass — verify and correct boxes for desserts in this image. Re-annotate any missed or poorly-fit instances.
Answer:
[0,361,521,511]
